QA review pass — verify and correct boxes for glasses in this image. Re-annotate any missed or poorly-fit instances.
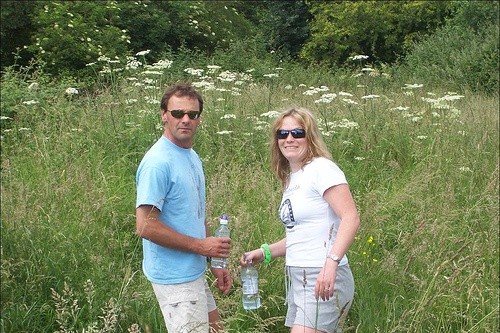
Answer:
[166,108,201,119]
[276,127,306,138]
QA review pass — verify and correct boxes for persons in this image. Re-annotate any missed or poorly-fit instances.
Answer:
[240,108,360,333]
[135,84,233,333]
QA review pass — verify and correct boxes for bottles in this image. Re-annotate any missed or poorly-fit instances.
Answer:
[240,254,260,310]
[211,214,230,269]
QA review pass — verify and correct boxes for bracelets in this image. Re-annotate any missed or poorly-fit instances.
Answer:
[261,243,271,265]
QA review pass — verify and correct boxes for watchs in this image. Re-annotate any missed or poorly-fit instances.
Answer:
[327,254,341,264]
